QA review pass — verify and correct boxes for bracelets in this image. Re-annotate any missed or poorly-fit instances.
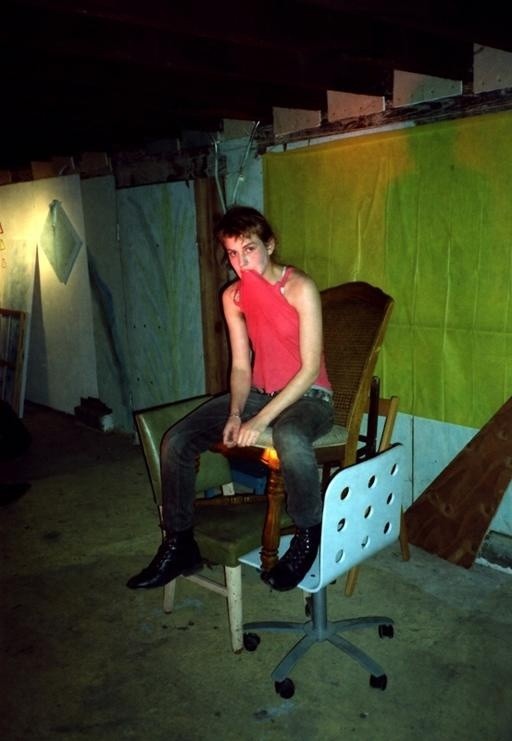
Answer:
[228,413,241,419]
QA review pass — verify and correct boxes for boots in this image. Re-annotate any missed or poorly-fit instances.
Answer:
[266,524,320,591]
[125,530,204,589]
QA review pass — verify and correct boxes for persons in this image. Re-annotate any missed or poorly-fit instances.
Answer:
[125,206,335,592]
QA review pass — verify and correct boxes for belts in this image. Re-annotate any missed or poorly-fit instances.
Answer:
[250,386,332,405]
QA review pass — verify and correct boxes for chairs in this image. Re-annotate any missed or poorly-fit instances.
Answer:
[231,443,404,697]
[314,375,404,598]
[208,281,391,579]
[134,393,292,655]
